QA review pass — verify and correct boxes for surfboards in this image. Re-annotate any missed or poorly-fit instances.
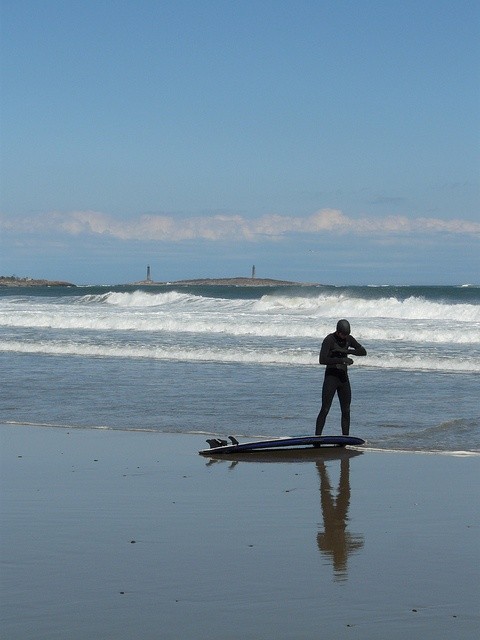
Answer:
[198,436,365,455]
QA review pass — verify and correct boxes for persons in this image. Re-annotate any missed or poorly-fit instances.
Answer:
[314,319,367,449]
